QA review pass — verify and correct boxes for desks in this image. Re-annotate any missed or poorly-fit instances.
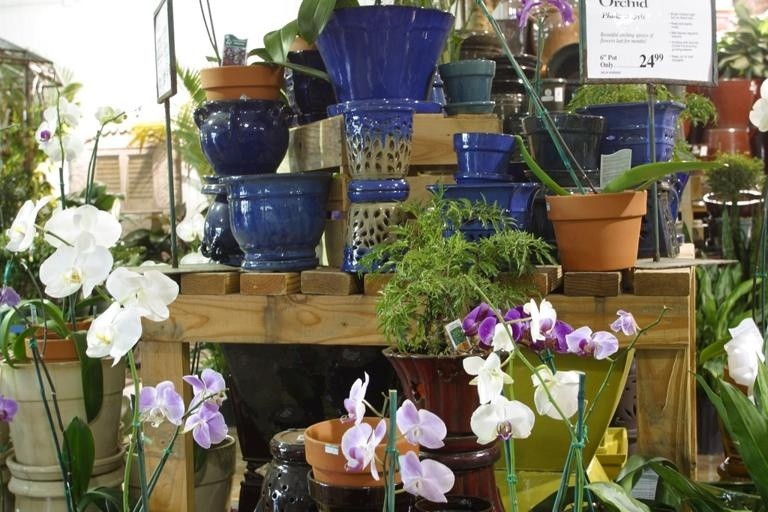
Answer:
[127,239,699,512]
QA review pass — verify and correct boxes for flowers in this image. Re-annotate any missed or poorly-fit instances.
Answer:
[0,192,231,512]
[462,301,681,512]
[342,371,456,512]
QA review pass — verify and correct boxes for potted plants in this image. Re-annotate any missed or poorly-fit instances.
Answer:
[0,288,113,424]
[358,173,558,453]
